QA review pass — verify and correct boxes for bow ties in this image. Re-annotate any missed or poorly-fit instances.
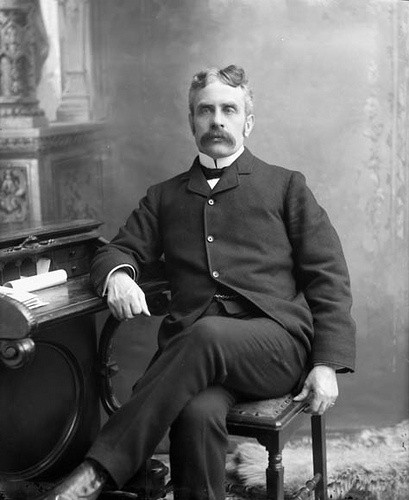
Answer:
[203,165,226,179]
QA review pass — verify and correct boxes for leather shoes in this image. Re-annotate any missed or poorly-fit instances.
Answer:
[43,460,106,500]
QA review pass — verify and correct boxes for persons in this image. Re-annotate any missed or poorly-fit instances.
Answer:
[43,66,357,500]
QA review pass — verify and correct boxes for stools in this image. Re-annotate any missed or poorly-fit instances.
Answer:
[226,392,328,499]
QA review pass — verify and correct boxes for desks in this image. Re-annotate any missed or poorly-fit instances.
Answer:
[0,219,171,500]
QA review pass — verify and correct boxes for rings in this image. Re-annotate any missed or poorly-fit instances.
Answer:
[331,403,335,407]
